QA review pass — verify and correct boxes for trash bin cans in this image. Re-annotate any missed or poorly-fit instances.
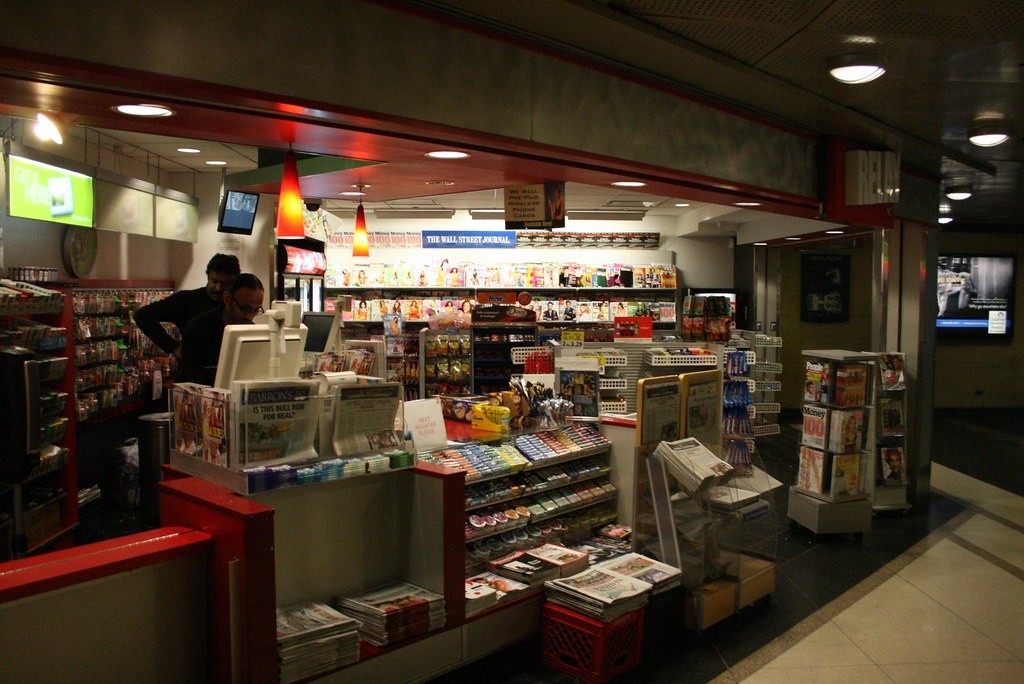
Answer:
[138,412,175,509]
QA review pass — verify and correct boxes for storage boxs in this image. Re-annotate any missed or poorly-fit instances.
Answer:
[539,603,645,684]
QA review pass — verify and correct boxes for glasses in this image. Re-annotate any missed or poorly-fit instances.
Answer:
[232,294,265,316]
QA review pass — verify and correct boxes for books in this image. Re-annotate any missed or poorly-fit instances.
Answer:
[324,258,681,324]
[877,355,906,392]
[274,575,448,684]
[465,523,685,615]
[169,381,233,469]
[880,399,906,438]
[794,358,867,499]
[880,448,909,486]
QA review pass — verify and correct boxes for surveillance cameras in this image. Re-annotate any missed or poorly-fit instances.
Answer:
[307,200,320,211]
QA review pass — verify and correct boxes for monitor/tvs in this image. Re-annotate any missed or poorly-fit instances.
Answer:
[935,253,1018,340]
[300,312,342,358]
[215,323,308,390]
[217,188,260,235]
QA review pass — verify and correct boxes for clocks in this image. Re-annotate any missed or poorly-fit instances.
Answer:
[62,226,98,279]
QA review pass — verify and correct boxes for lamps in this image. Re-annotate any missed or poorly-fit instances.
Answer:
[37,110,67,145]
[275,144,306,239]
[937,214,954,224]
[944,185,974,201]
[353,187,370,258]
[966,125,1013,148]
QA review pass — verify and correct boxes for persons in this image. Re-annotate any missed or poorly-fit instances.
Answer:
[357,301,368,321]
[885,448,903,485]
[444,300,454,312]
[470,269,480,286]
[379,301,389,319]
[457,299,472,323]
[564,300,576,320]
[357,270,366,286]
[616,303,625,317]
[841,415,858,450]
[803,381,815,400]
[471,578,521,600]
[408,301,420,320]
[366,432,398,451]
[543,302,558,320]
[179,399,191,452]
[186,405,196,452]
[201,400,210,461]
[217,404,226,465]
[172,273,267,388]
[419,270,427,286]
[342,270,351,286]
[598,300,606,320]
[174,395,183,450]
[448,268,459,288]
[208,405,219,463]
[881,355,904,389]
[636,309,643,317]
[426,300,439,319]
[131,251,242,362]
[883,406,902,428]
[389,299,403,315]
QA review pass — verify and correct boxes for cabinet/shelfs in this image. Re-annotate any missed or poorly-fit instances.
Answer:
[0,250,912,684]
